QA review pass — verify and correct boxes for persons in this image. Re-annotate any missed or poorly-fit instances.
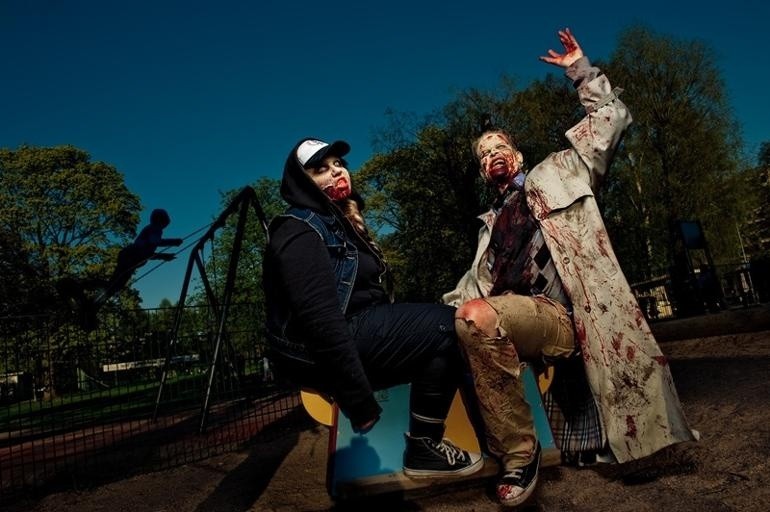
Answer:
[439,26,697,511]
[81,207,184,293]
[259,135,485,483]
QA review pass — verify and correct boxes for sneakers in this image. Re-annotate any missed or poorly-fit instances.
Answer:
[403,431,485,480]
[495,440,542,507]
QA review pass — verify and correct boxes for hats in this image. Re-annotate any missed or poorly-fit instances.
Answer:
[296,139,350,170]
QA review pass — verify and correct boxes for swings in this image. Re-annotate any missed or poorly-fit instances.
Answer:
[56,205,239,328]
